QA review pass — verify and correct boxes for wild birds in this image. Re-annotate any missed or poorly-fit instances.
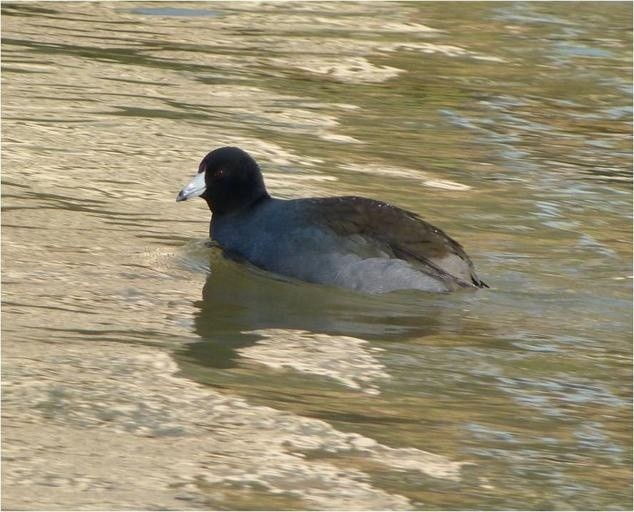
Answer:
[175,146,490,294]
